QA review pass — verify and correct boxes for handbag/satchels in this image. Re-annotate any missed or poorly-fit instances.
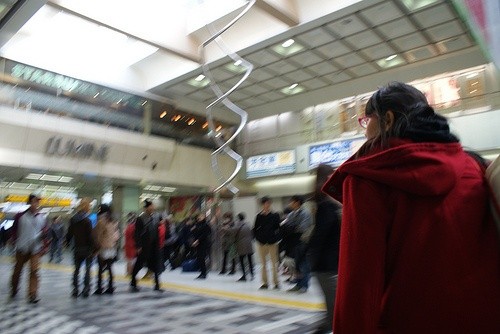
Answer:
[97,254,120,274]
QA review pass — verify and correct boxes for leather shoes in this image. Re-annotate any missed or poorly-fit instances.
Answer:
[288,284,308,293]
[91,287,116,295]
[259,284,269,290]
[71,285,90,298]
[274,286,280,289]
[238,275,246,281]
[229,271,235,275]
[218,270,227,274]
[197,272,208,279]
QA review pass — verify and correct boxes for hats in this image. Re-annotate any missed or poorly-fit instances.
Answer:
[261,196,272,204]
[96,204,113,222]
[144,200,152,207]
[26,194,42,205]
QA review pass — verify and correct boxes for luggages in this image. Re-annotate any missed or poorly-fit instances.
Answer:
[182,257,199,272]
[168,242,192,270]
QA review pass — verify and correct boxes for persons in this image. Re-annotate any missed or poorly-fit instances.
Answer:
[208,204,254,282]
[63,200,96,298]
[125,198,212,293]
[0,216,65,264]
[10,194,49,303]
[320,81,500,334]
[294,163,342,334]
[254,163,342,294]
[91,204,120,296]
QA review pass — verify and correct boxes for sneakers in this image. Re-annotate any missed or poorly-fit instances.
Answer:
[152,289,163,293]
[129,286,138,293]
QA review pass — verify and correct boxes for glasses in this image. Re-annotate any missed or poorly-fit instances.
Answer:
[358,114,377,129]
